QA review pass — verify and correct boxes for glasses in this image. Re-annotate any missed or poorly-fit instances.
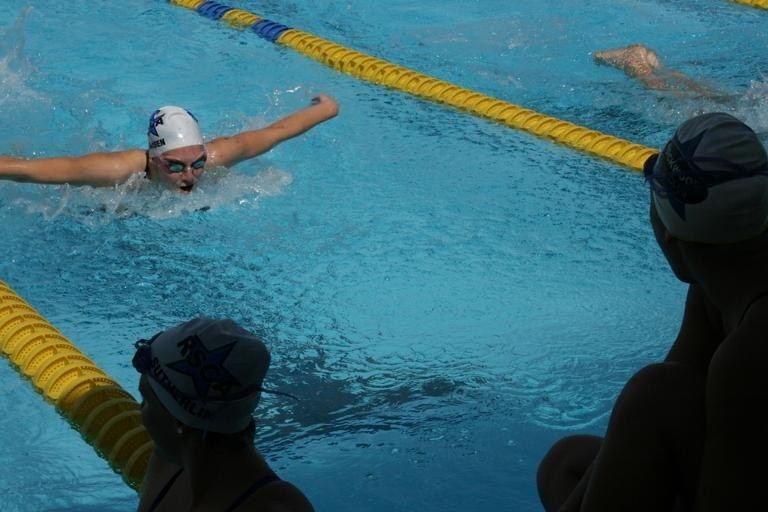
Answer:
[154,160,207,172]
[132,332,259,418]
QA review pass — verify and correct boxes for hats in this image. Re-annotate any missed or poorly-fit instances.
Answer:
[148,107,204,158]
[144,317,270,433]
[651,112,767,243]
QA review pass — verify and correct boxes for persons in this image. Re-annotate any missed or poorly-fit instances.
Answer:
[594,44,741,107]
[131,316,314,511]
[1,92,339,194]
[535,112,768,512]
